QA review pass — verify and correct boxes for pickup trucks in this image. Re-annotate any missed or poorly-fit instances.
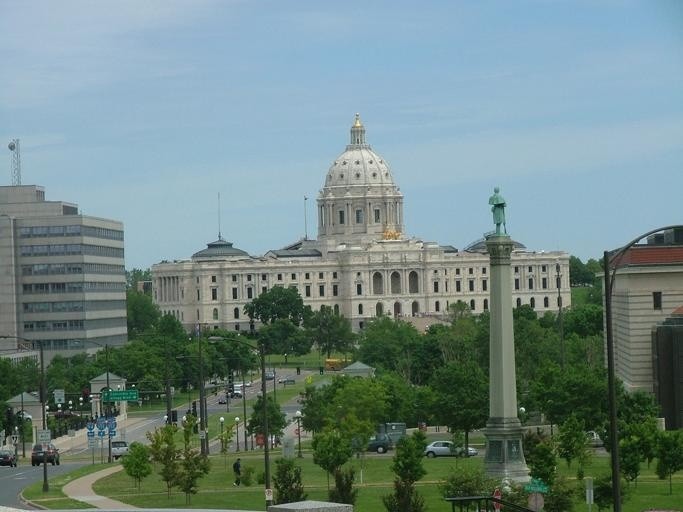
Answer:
[112,441,131,460]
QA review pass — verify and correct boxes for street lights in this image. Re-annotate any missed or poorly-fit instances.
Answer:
[208,335,275,512]
[235,417,240,453]
[219,417,225,449]
[71,338,113,465]
[294,411,305,459]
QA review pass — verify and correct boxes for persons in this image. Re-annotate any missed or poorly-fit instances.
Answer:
[488,187,508,234]
[232,457,241,487]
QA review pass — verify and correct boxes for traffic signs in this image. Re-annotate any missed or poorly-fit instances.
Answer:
[102,389,139,402]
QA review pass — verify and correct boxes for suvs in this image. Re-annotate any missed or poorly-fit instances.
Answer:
[365,433,392,455]
[30,443,60,467]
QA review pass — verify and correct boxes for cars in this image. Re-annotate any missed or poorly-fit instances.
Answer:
[423,440,479,458]
[0,450,17,469]
